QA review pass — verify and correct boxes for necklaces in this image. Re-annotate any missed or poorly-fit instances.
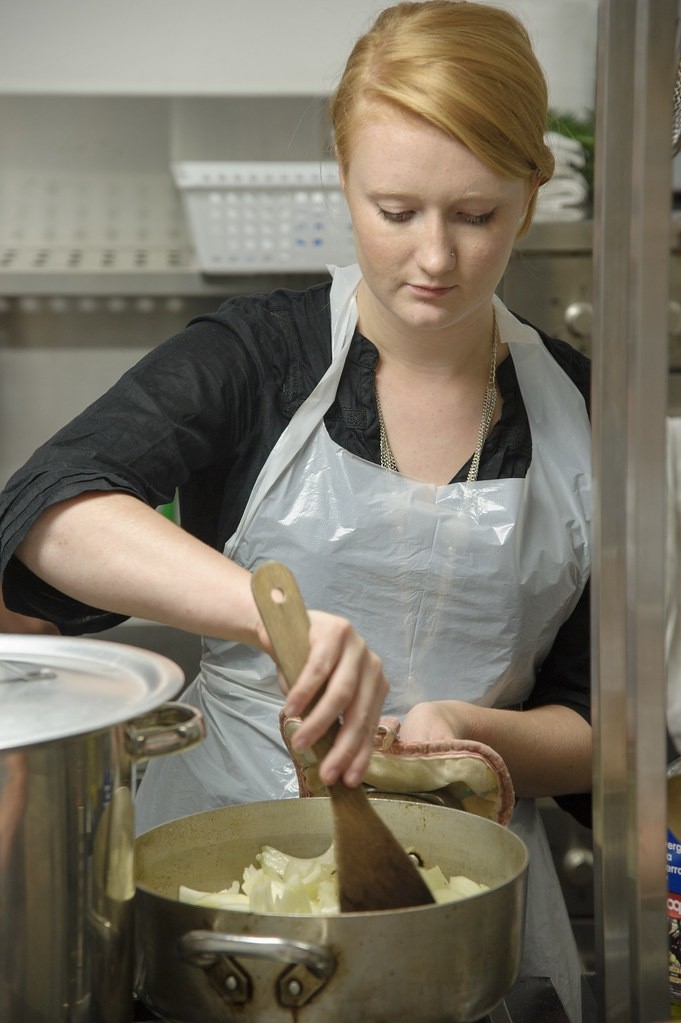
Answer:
[351,297,506,488]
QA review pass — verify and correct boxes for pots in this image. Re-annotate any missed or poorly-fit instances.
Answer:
[0,633,206,1023]
[132,798,530,1022]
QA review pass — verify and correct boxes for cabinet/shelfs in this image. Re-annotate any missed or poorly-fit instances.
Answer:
[0,0,598,322]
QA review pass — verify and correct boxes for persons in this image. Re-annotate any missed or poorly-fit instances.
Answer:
[0,2,614,1021]
